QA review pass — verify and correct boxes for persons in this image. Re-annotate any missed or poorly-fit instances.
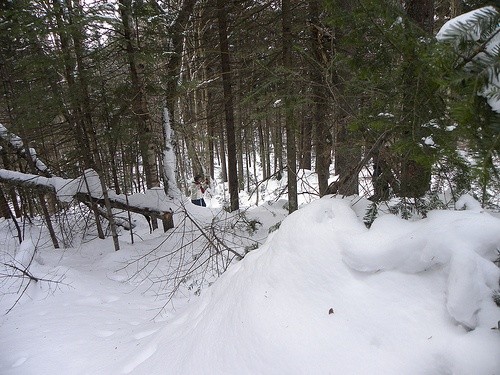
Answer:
[190,175,209,207]
